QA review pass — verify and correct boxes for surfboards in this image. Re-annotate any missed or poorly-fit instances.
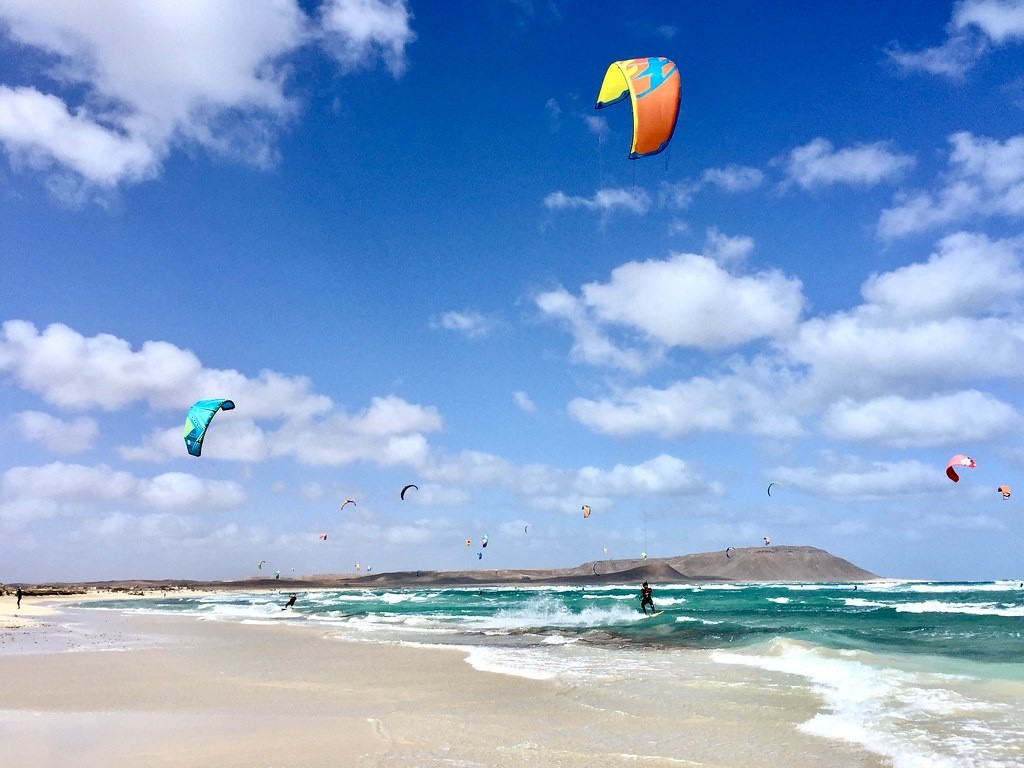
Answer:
[282,608,287,611]
[650,610,665,619]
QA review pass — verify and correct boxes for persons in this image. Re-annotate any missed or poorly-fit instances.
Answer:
[640,580,656,616]
[581,586,584,592]
[281,593,297,611]
[853,585,858,591]
[15,586,23,609]
[477,587,482,595]
